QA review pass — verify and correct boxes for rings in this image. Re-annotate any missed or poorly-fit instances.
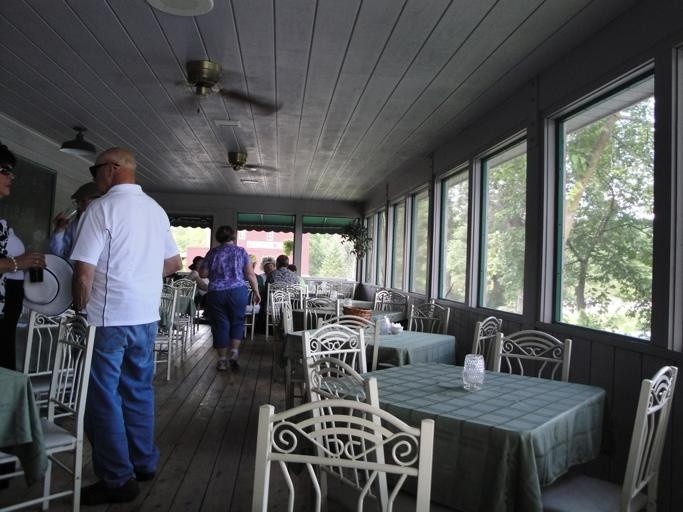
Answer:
[33,259,40,264]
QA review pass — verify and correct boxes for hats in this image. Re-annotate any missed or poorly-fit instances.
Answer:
[71,182,102,200]
[21,253,76,317]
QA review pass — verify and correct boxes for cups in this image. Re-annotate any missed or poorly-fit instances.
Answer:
[311,281,353,309]
[28,253,43,284]
[53,206,79,228]
[462,354,485,394]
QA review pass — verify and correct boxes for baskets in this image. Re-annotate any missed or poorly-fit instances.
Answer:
[342,306,371,326]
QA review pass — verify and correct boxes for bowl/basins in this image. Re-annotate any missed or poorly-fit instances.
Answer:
[342,306,373,324]
[390,327,404,335]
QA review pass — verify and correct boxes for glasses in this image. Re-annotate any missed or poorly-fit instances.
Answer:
[89,162,120,178]
[0,166,19,175]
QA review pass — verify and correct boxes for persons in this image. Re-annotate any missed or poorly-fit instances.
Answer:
[50,180,105,266]
[258,256,277,288]
[197,224,262,372]
[265,254,306,314]
[242,254,264,306]
[184,255,210,309]
[69,143,183,507]
[286,263,306,290]
[0,138,49,369]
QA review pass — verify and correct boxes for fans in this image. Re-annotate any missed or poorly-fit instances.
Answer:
[212,151,280,176]
[109,58,284,119]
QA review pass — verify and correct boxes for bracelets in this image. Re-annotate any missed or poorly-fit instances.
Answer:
[6,255,19,273]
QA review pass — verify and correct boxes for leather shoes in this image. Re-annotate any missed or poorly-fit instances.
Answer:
[136,470,155,481]
[81,476,140,506]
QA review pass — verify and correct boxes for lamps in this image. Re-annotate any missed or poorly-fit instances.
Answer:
[59,126,96,157]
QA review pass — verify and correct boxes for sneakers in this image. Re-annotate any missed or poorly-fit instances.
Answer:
[229,355,239,369]
[215,358,227,371]
